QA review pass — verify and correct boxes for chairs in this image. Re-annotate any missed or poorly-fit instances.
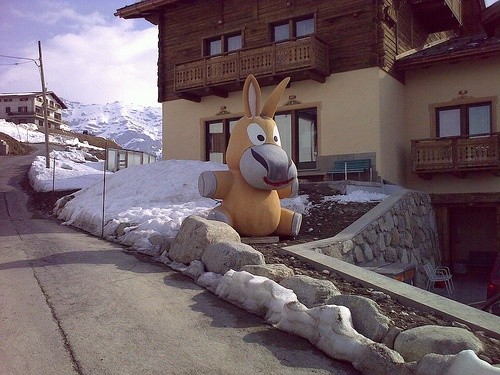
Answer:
[427,262,454,291]
[423,264,452,296]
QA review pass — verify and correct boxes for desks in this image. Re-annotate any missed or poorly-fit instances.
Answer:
[375,262,417,287]
[360,261,394,271]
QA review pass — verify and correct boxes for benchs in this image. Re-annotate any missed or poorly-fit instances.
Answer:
[327,159,373,181]
